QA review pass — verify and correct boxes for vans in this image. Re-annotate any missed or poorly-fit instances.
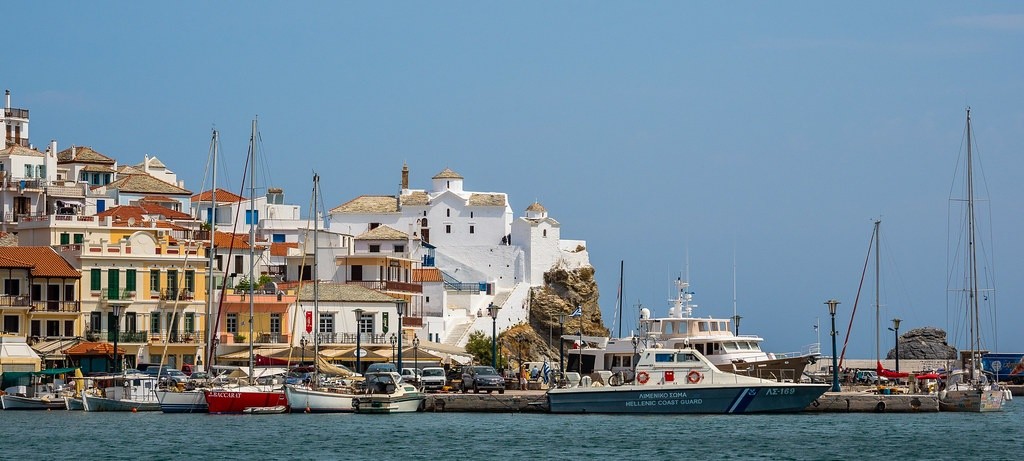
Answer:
[420,368,447,392]
[513,361,561,378]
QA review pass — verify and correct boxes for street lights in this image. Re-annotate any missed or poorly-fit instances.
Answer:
[351,308,365,374]
[820,298,842,392]
[485,301,503,369]
[558,314,568,389]
[515,333,525,391]
[497,337,505,377]
[107,304,125,375]
[393,298,409,374]
[888,318,903,373]
[412,332,421,386]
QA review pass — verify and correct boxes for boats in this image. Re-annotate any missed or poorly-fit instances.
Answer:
[731,315,743,337]
[526,244,834,418]
[0,292,215,417]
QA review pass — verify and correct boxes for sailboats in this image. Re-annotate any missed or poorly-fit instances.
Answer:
[279,168,432,415]
[940,104,1013,414]
[196,116,291,416]
[839,214,942,396]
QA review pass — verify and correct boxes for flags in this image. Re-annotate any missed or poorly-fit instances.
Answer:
[569,305,581,317]
[544,361,550,384]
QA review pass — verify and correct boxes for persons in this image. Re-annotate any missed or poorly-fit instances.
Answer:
[532,366,538,377]
[521,364,530,390]
[841,366,862,386]
[499,365,504,376]
[510,366,515,378]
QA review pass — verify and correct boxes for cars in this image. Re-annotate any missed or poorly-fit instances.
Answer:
[285,366,314,384]
[185,372,216,390]
[459,366,506,395]
[364,363,397,384]
[445,365,472,393]
[146,366,188,383]
[401,368,423,384]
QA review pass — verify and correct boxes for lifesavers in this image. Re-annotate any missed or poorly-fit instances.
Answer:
[169,378,178,386]
[123,379,130,388]
[688,371,701,383]
[877,402,886,411]
[637,372,649,383]
